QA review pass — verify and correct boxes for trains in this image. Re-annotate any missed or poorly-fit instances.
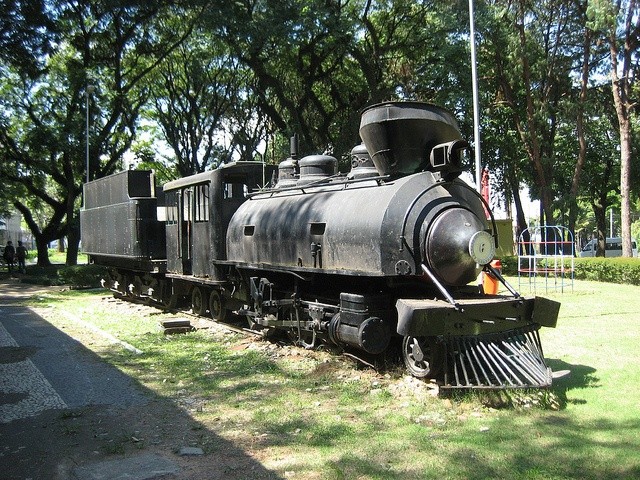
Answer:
[79,101,561,390]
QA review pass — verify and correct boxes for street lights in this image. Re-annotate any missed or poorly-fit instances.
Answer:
[83,80,96,183]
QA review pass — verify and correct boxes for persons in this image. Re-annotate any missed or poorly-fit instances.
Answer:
[5,241,15,272]
[16,241,28,270]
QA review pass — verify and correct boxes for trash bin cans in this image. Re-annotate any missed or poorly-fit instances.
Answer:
[482,259,502,294]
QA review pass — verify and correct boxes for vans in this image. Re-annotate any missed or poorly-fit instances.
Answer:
[580,237,638,257]
